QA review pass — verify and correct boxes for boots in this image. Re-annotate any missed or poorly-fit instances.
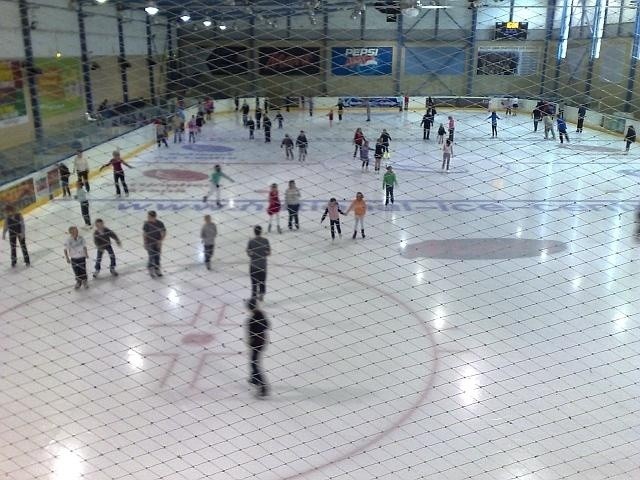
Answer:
[353,230,357,238]
[361,229,366,238]
[277,225,282,233]
[268,225,272,232]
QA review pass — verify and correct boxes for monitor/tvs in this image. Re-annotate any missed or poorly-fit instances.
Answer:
[495,22,528,41]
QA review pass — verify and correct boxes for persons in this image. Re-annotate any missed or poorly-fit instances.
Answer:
[623,125,636,153]
[245,298,270,398]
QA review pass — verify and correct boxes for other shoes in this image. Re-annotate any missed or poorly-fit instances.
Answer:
[217,203,223,207]
[286,156,306,162]
[423,137,444,144]
[202,196,207,202]
[353,152,390,171]
[68,193,71,197]
[117,193,121,198]
[63,194,66,197]
[580,129,582,133]
[86,188,89,192]
[490,136,498,139]
[385,202,389,206]
[622,151,628,155]
[534,129,570,145]
[155,130,201,148]
[125,192,130,198]
[391,198,394,203]
[442,164,450,170]
[576,129,579,132]
[241,124,284,144]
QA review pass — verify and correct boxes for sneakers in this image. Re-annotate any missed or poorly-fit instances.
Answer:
[11,257,18,267]
[74,277,88,288]
[289,224,299,229]
[92,269,100,278]
[149,266,163,278]
[23,256,30,264]
[109,266,118,276]
[205,261,211,270]
[250,378,271,396]
[258,294,264,301]
[81,224,93,229]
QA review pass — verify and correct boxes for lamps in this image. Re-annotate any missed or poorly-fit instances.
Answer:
[402,8,419,19]
[95,0,366,34]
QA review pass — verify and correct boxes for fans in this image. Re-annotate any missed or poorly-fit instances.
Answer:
[372,0,451,12]
